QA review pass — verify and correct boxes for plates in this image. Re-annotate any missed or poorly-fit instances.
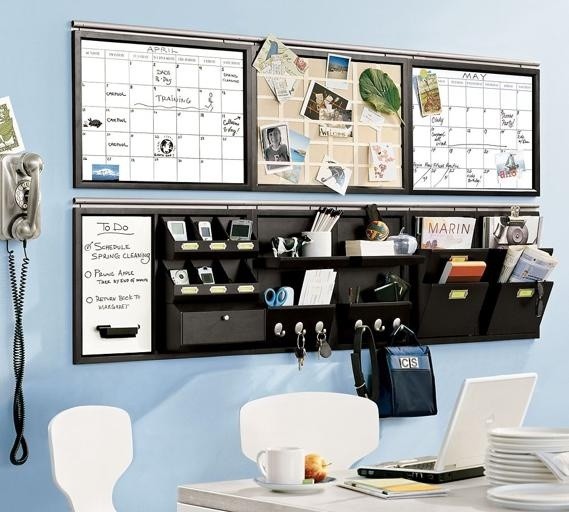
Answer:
[254,476,337,494]
[483,428,569,512]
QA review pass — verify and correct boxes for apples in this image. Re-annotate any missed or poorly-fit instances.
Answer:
[306,455,333,483]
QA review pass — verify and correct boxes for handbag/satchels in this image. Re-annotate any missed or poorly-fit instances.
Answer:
[370,323,437,418]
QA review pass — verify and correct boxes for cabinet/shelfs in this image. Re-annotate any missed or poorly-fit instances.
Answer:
[257,211,416,348]
[154,213,267,356]
[417,213,552,344]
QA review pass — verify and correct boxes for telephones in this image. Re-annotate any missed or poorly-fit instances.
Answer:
[0,149,46,245]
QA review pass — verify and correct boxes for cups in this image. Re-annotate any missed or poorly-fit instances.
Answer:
[256,447,305,485]
[302,231,331,257]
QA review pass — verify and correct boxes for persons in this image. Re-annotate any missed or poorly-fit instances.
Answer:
[264,128,290,170]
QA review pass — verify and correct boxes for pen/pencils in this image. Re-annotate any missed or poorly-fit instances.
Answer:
[354,286,361,303]
[311,206,343,232]
[348,288,352,305]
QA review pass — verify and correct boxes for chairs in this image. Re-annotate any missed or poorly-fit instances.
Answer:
[239,391,381,478]
[45,400,134,512]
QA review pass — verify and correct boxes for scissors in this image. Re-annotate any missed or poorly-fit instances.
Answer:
[264,287,287,307]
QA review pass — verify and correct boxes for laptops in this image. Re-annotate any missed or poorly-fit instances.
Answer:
[356,372,538,485]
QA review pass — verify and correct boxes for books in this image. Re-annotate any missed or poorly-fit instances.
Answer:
[439,260,487,284]
[415,215,476,250]
[482,216,540,248]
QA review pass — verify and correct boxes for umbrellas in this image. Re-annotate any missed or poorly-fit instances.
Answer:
[321,161,345,189]
